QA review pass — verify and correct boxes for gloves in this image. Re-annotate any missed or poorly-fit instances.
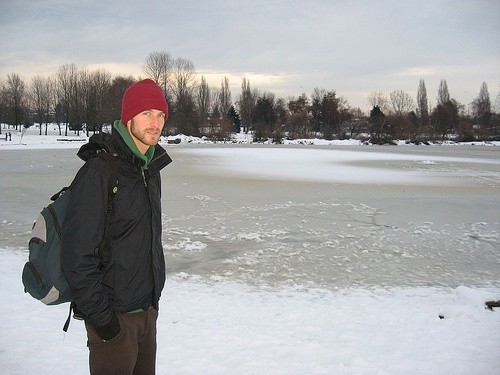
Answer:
[99,319,120,340]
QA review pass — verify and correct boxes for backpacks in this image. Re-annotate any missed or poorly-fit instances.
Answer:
[22,153,120,306]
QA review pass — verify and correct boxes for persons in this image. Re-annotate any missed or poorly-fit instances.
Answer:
[60,79,169,375]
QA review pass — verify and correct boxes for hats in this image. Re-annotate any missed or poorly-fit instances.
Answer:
[121,80,169,125]
